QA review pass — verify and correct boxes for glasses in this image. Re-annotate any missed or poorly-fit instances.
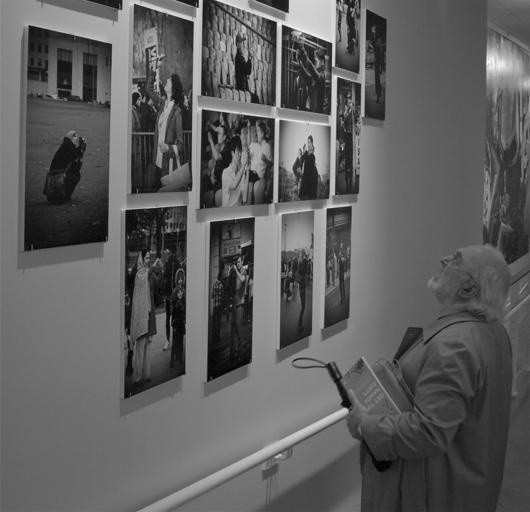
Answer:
[452,250,477,281]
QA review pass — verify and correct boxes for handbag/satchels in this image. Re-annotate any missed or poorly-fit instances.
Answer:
[160,144,191,187]
[43,161,73,197]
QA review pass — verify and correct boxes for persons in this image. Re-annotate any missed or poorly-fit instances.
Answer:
[229,254,248,351]
[132,92,141,193]
[246,118,274,205]
[129,249,159,388]
[333,247,346,305]
[291,35,327,114]
[337,10,342,42]
[221,136,250,207]
[293,73,307,109]
[159,245,181,351]
[208,112,230,132]
[294,248,308,333]
[43,129,88,206]
[169,286,186,368]
[347,241,514,512]
[202,126,234,208]
[235,31,260,104]
[240,117,258,146]
[211,270,225,344]
[146,60,185,192]
[372,31,382,103]
[292,134,318,201]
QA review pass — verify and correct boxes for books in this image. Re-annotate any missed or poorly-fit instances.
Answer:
[339,356,402,416]
